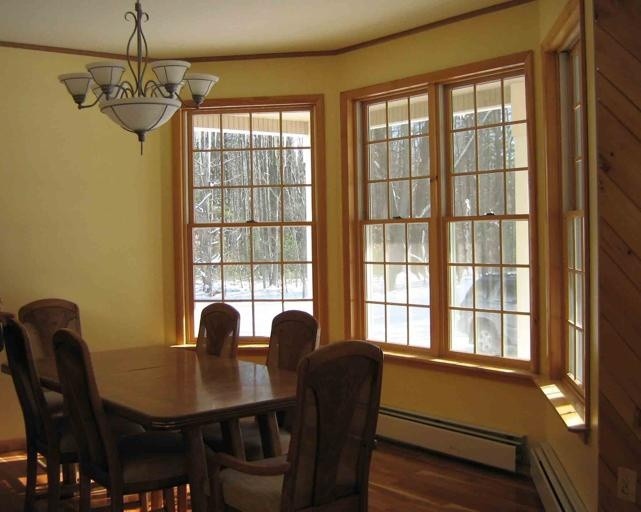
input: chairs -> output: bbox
[53,327,222,512]
[207,338,384,512]
[206,310,320,461]
[57,0,219,156]
[201,303,241,431]
[3,318,146,512]
[19,298,81,415]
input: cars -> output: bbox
[458,272,517,356]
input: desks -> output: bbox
[1,343,300,511]
